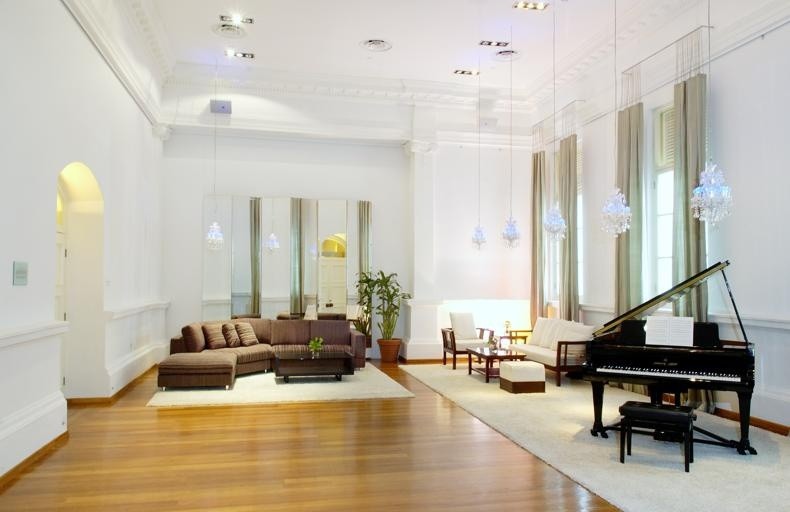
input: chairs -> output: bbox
[440,325,496,372]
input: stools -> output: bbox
[497,360,548,394]
[613,394,698,474]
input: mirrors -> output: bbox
[227,194,375,350]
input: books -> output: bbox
[644,317,694,347]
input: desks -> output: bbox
[498,334,528,348]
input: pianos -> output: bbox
[585,261,757,455]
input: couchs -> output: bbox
[156,315,367,391]
[509,328,595,388]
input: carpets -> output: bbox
[144,360,419,409]
[396,357,789,512]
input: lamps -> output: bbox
[540,0,571,245]
[690,0,735,227]
[263,194,283,253]
[472,1,491,248]
[500,0,524,248]
[597,0,636,238]
[202,60,231,252]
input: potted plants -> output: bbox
[350,270,372,348]
[370,269,413,363]
[484,335,499,350]
[308,334,324,360]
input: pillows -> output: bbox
[234,321,261,347]
[202,323,228,350]
[527,315,596,355]
[221,323,240,348]
[449,311,480,340]
